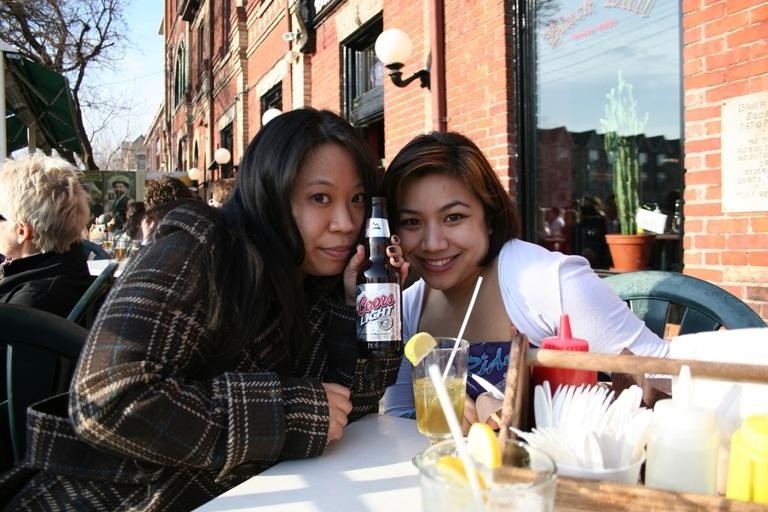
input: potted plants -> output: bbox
[599,71,656,271]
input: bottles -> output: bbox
[532,313,599,393]
[644,362,720,496]
[726,413,768,507]
[353,195,405,363]
[671,199,682,234]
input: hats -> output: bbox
[108,176,131,186]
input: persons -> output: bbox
[0,150,94,474]
[0,104,410,511]
[380,128,670,436]
[540,189,681,275]
[80,174,236,260]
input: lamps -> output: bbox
[187,27,430,188]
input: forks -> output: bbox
[507,380,653,471]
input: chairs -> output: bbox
[604,271,765,340]
[66,263,119,322]
[0,303,90,482]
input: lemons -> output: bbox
[404,332,438,367]
[437,421,503,504]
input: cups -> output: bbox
[408,336,471,438]
[103,240,139,261]
[411,434,558,512]
